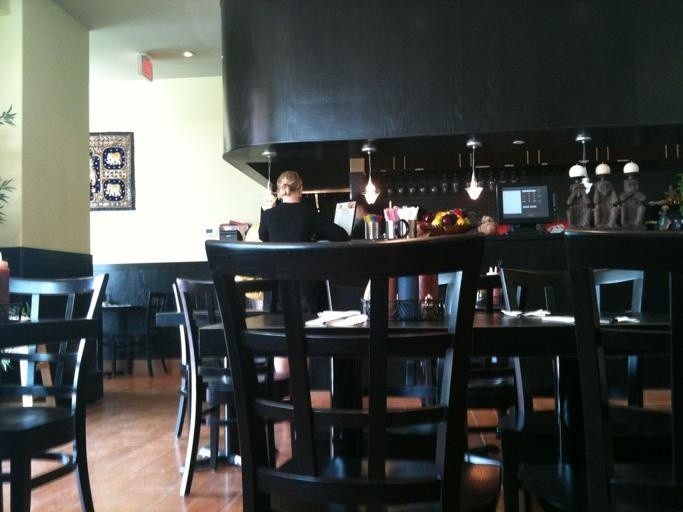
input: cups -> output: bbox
[364,220,410,241]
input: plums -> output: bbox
[442,215,452,226]
[422,213,433,222]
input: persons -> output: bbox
[344,192,384,238]
[257,170,349,399]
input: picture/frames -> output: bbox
[87,131,136,211]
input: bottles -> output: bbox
[381,170,521,194]
[565,175,645,229]
[362,273,437,321]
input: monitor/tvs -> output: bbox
[495,181,553,236]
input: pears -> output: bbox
[432,217,442,226]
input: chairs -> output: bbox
[173,230,683,512]
[110,292,173,377]
[1,273,110,512]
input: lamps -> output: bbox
[361,140,379,205]
[464,139,483,201]
[566,132,640,182]
[258,150,277,210]
